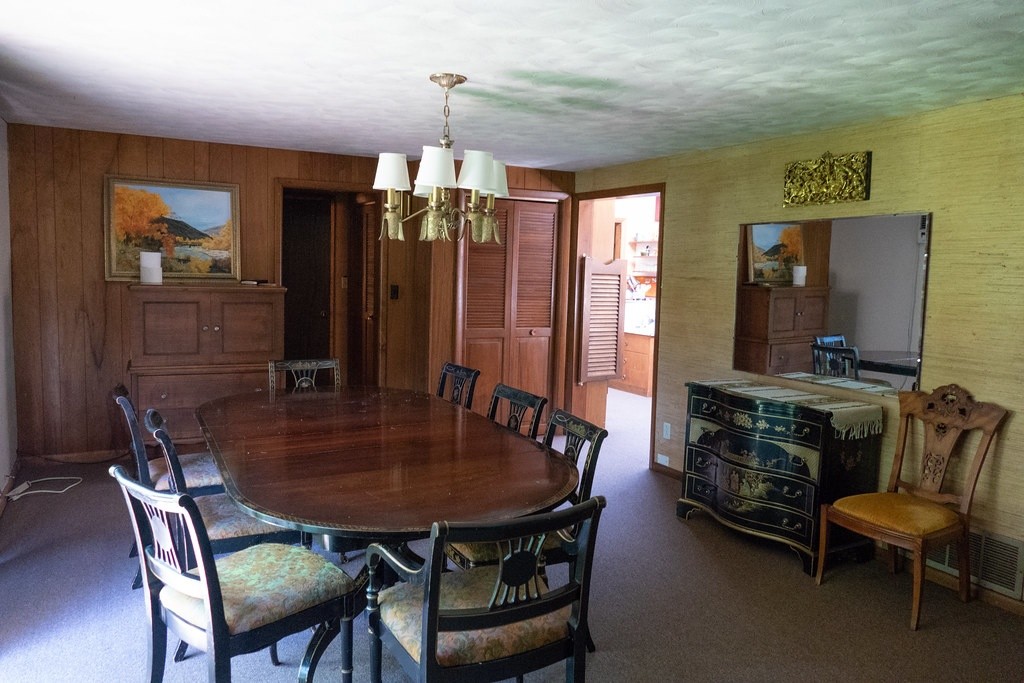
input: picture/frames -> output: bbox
[103,172,242,284]
[748,223,804,282]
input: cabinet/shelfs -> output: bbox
[733,220,832,375]
[608,241,657,398]
[677,380,884,581]
[125,282,287,445]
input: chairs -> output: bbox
[108,361,610,682]
[816,383,1009,632]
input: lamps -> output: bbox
[373,72,507,246]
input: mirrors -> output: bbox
[731,211,932,393]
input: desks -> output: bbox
[194,384,578,683]
